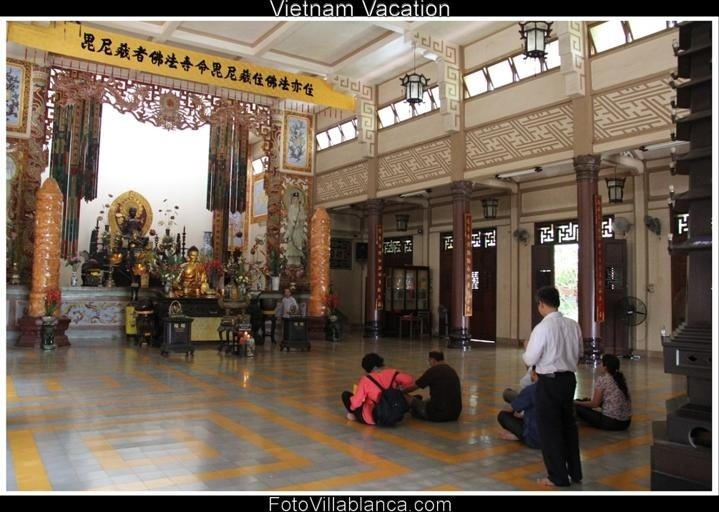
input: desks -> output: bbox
[261,315,278,344]
[280,316,312,352]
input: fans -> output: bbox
[612,217,632,237]
[614,295,647,360]
[513,228,532,246]
[643,214,663,235]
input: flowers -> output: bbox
[41,288,62,317]
[205,258,224,278]
[112,246,155,284]
[323,291,341,313]
[65,255,82,272]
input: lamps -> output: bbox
[518,21,554,59]
[395,212,410,232]
[605,165,627,203]
[400,44,430,105]
[482,194,499,220]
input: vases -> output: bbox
[124,284,140,335]
[71,272,78,287]
[42,316,57,349]
[327,313,343,341]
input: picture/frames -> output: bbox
[279,106,315,178]
[6,57,33,139]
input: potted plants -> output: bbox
[267,248,287,291]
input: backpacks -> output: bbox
[366,371,408,428]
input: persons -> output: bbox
[173,244,217,299]
[410,350,463,423]
[121,205,150,246]
[521,286,584,486]
[275,287,301,353]
[282,189,307,267]
[497,364,542,451]
[341,352,417,425]
[574,353,631,431]
